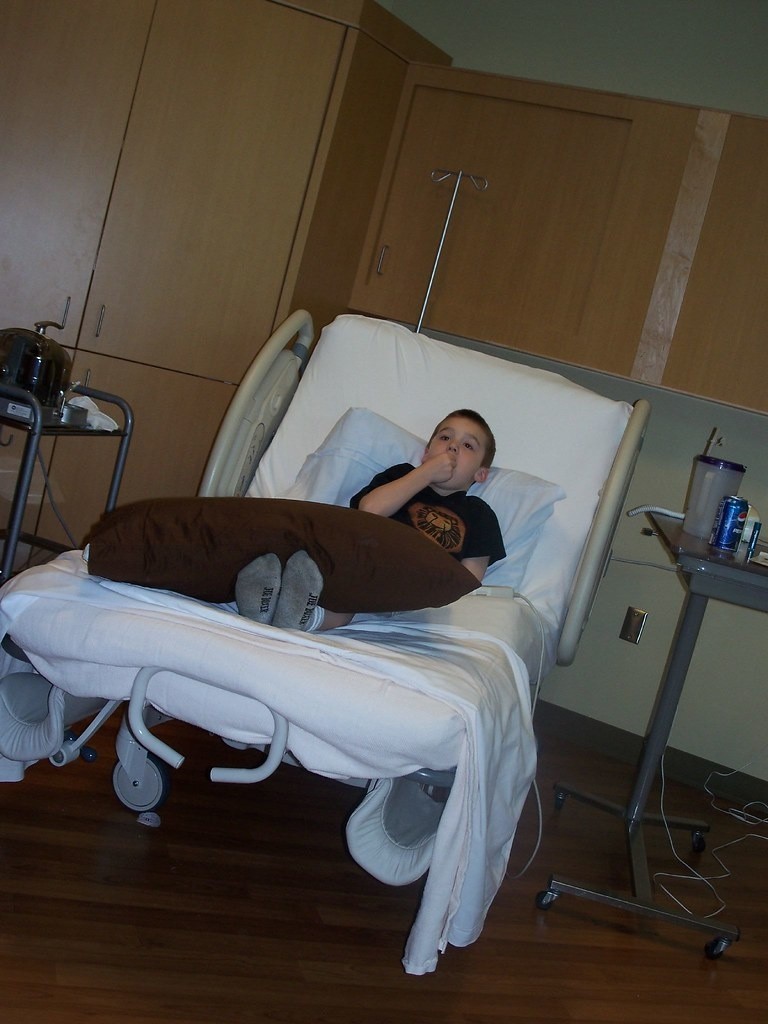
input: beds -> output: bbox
[0,309,650,975]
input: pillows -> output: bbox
[78,499,482,612]
[295,408,567,592]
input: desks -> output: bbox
[537,509,767,958]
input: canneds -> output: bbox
[708,495,749,552]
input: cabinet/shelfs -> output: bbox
[0,0,768,589]
[0,382,135,575]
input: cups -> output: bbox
[682,454,748,541]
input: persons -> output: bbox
[235,409,508,631]
